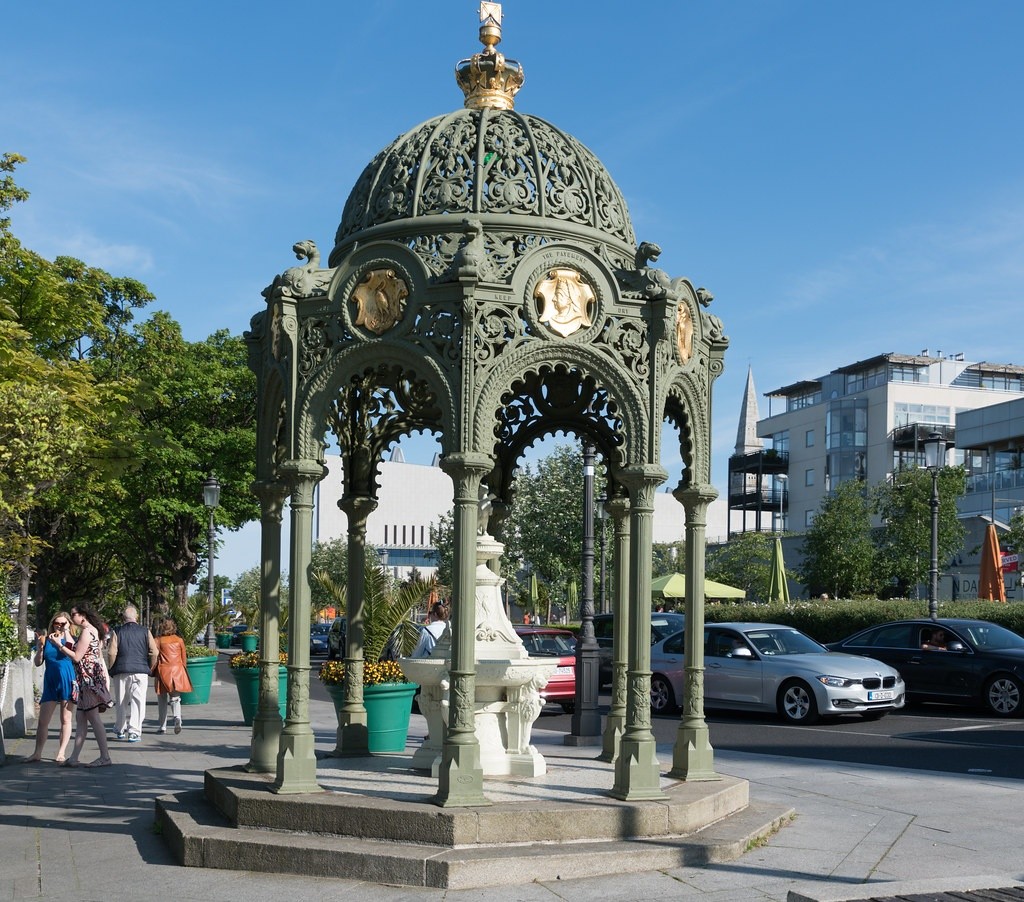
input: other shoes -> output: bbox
[157,730,165,734]
[174,717,181,734]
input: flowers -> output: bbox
[261,596,289,639]
[161,589,231,658]
[227,593,296,668]
[214,608,236,635]
[309,560,439,685]
[237,605,261,636]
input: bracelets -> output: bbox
[60,646,63,651]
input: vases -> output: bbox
[280,637,288,652]
[242,635,257,652]
[218,634,231,648]
[231,667,288,724]
[178,654,219,703]
[326,682,419,752]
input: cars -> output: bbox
[576,613,685,692]
[650,623,906,726]
[230,625,247,646]
[310,623,331,655]
[327,617,346,661]
[514,627,579,714]
[824,619,1024,718]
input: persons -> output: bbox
[19,611,76,763]
[820,594,828,601]
[922,629,947,650]
[153,617,193,734]
[49,601,116,768]
[411,598,452,743]
[655,605,663,612]
[523,611,535,624]
[552,613,556,625]
[107,607,159,742]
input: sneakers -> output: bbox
[128,733,140,742]
[114,727,125,738]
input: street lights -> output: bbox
[922,426,949,618]
[379,548,390,593]
[205,472,221,651]
[775,474,788,535]
[595,491,610,614]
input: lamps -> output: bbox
[952,553,963,566]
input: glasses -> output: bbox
[70,612,78,618]
[53,622,65,626]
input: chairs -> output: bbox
[542,639,557,650]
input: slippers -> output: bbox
[84,759,111,767]
[21,756,40,763]
[59,758,78,767]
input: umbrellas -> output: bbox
[978,522,1005,603]
[524,570,539,616]
[766,538,790,604]
[427,580,438,610]
[567,571,578,609]
[651,572,746,608]
